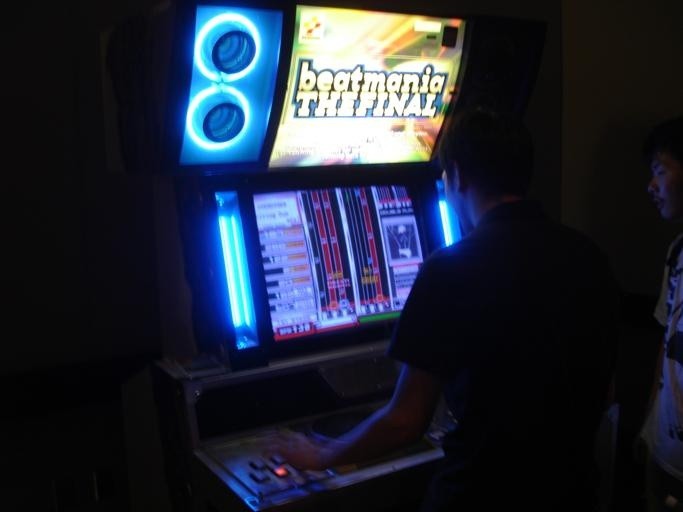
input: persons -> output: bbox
[630,116,682,510]
[269,106,642,510]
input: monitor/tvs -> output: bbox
[254,185,423,341]
